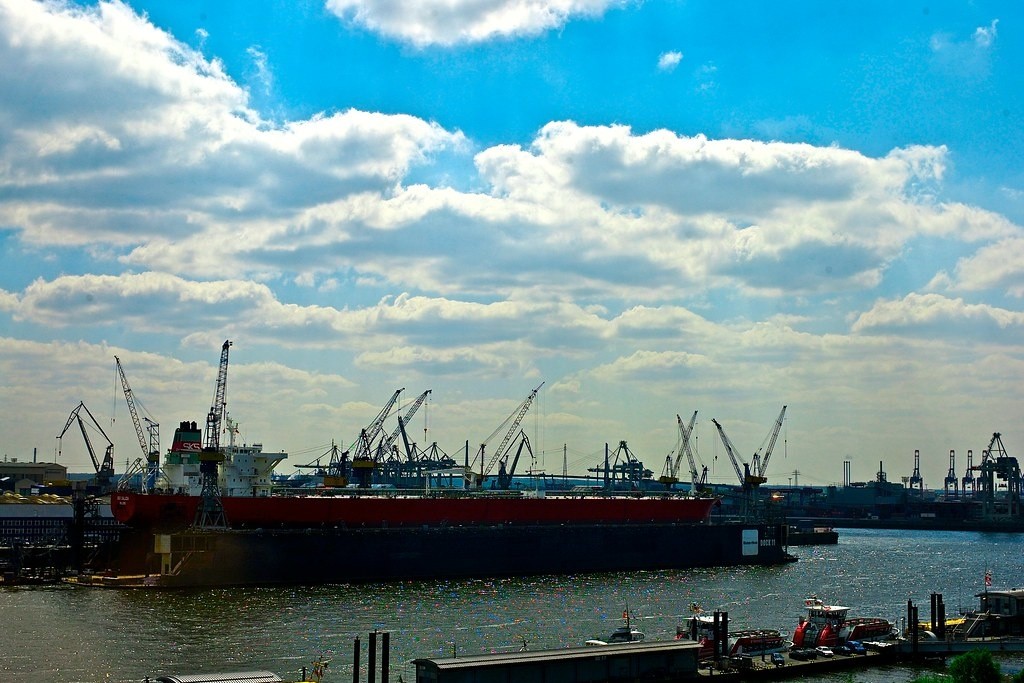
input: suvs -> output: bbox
[847,641,866,655]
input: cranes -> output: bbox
[290,387,432,488]
[108,338,241,554]
[458,381,544,493]
[712,403,789,525]
[55,401,116,500]
[658,409,711,498]
[907,432,1024,499]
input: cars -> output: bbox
[771,652,787,667]
[832,646,852,657]
[790,647,818,660]
[816,646,834,658]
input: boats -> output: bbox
[790,596,900,653]
[586,601,646,649]
[674,601,793,664]
[905,567,1024,641]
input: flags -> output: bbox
[985,567,994,586]
